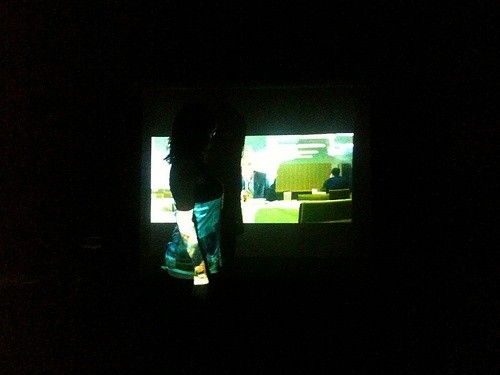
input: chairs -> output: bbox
[329,188,351,199]
[298,200,352,223]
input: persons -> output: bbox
[161,101,223,298]
[323,168,345,193]
